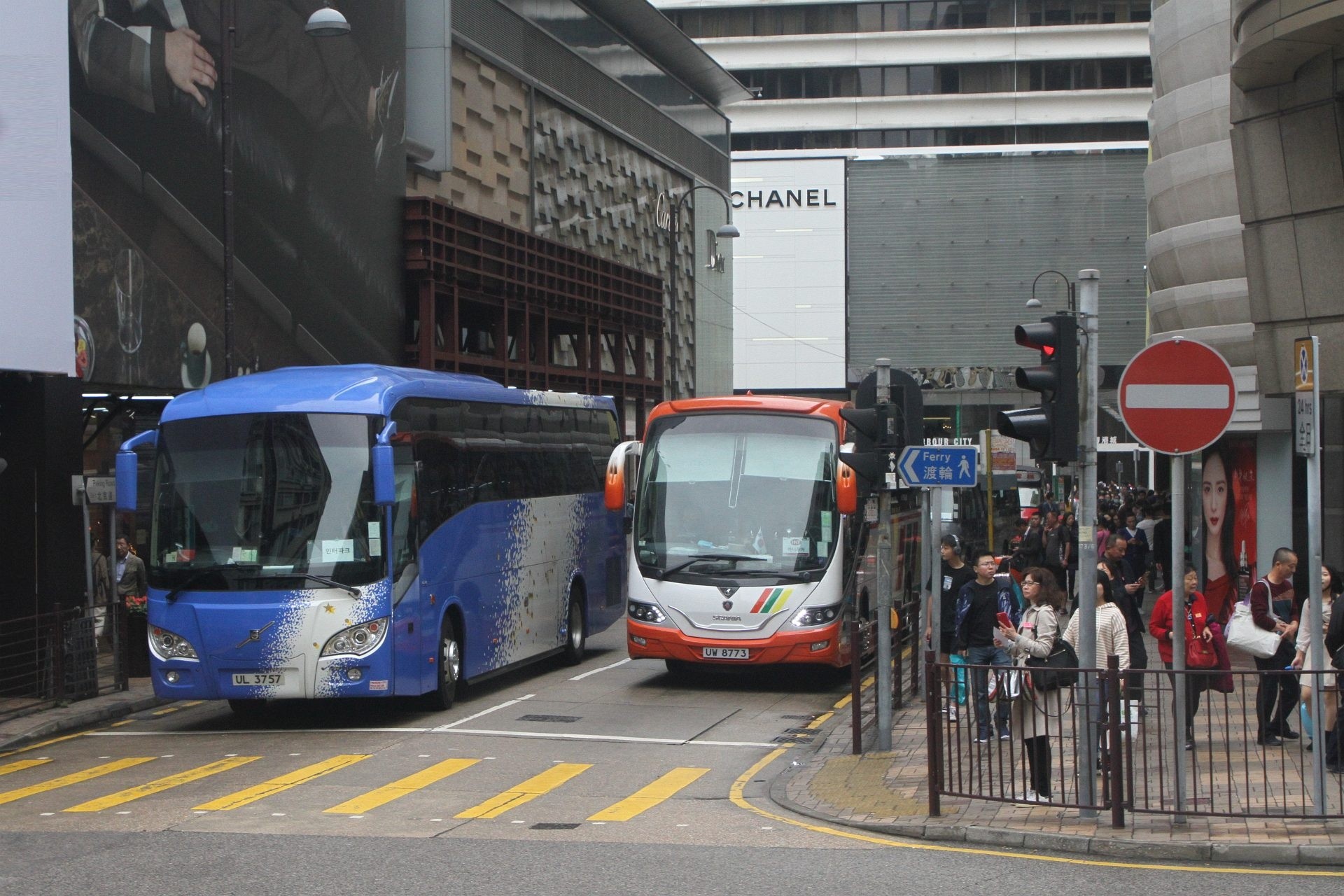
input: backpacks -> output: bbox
[88,553,103,605]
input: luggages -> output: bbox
[1284,666,1313,741]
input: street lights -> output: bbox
[669,182,742,400]
[1026,269,1079,514]
[217,0,354,377]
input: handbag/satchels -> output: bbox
[987,673,997,704]
[1145,550,1156,571]
[1025,604,1080,692]
[1223,578,1283,659]
[1119,699,1139,742]
[1057,525,1071,566]
[1187,637,1219,668]
[949,654,970,707]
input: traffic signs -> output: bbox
[896,445,979,487]
[86,476,115,504]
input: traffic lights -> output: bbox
[995,312,1078,469]
[837,403,906,492]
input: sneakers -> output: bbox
[1077,758,1101,777]
[1010,786,1036,805]
[1016,789,1053,808]
[949,706,956,722]
[1101,759,1111,780]
[973,732,995,743]
[942,702,947,713]
[1000,727,1010,741]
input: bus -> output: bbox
[114,364,633,715]
[604,388,920,686]
[926,465,1048,566]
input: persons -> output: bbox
[626,490,636,507]
[69,0,404,321]
[1200,447,1239,627]
[90,536,151,676]
[925,481,1344,808]
[1149,561,1234,749]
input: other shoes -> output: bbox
[1257,738,1281,746]
[1185,738,1192,750]
[1307,743,1313,751]
[1272,728,1299,739]
[1139,606,1147,614]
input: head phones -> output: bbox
[952,534,962,555]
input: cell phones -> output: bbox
[997,612,1011,628]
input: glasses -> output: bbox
[976,563,997,567]
[1021,580,1041,586]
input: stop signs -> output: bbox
[1115,336,1239,457]
